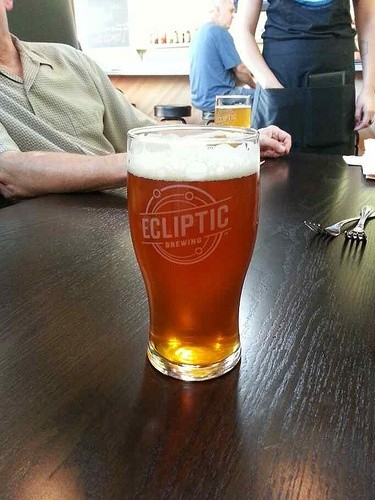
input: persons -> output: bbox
[0,0,291,210]
[190,0,257,113]
[233,0,375,148]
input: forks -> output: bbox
[304,211,375,237]
[344,205,373,240]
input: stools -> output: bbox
[154,105,191,124]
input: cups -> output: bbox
[126,125,260,381]
[214,95,251,128]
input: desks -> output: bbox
[0,153,375,500]
